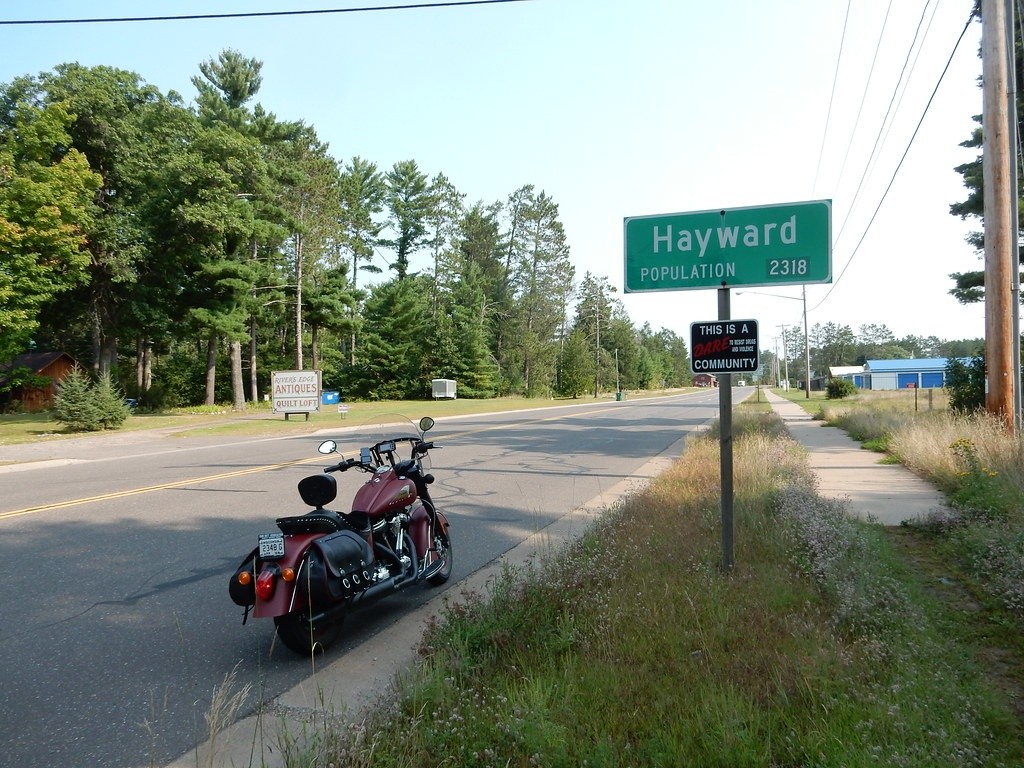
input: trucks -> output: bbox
[737,381,745,387]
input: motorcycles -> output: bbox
[252,412,453,659]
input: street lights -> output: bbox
[735,290,810,399]
